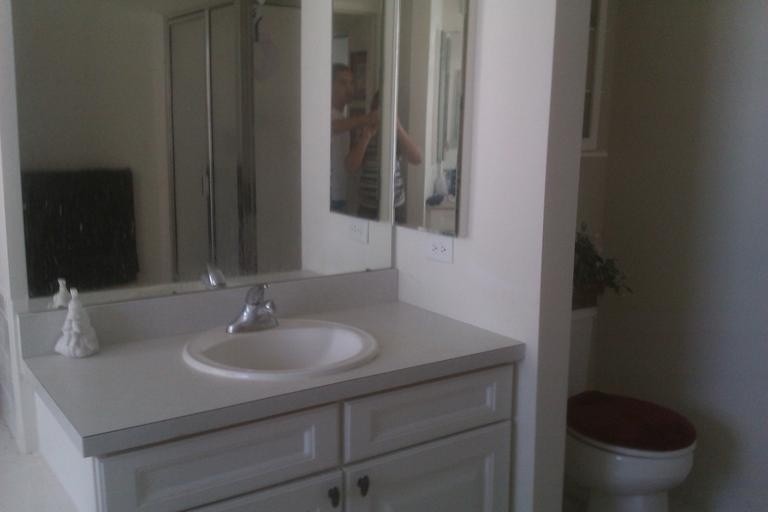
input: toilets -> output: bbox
[566,302,701,511]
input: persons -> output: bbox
[331,62,380,222]
[342,92,423,226]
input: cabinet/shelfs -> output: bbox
[97,361,516,511]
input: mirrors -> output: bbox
[393,1,470,238]
[12,1,395,313]
[328,1,392,221]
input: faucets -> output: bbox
[202,256,228,288]
[222,279,280,332]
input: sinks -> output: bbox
[181,313,380,383]
